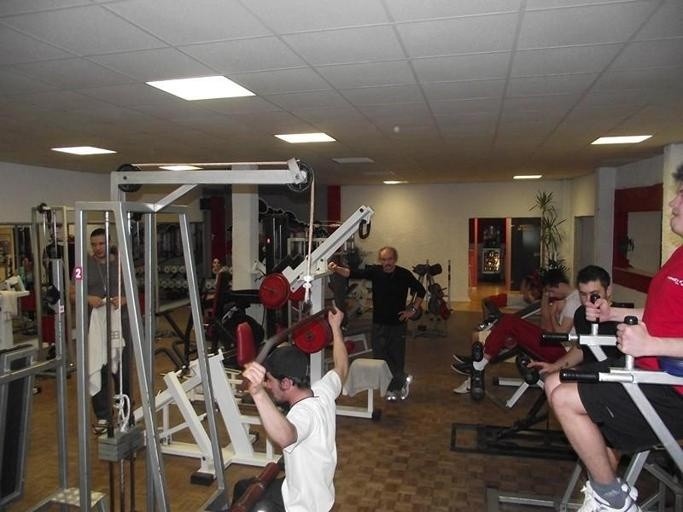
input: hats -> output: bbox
[260,345,310,384]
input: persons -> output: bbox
[328,247,426,401]
[65,227,132,434]
[541,161,683,511]
[449,269,583,375]
[15,256,42,282]
[208,255,233,289]
[526,264,632,375]
[231,308,348,512]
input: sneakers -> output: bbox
[453,353,474,363]
[575,479,642,512]
[450,362,474,375]
[92,419,109,435]
[400,373,413,400]
[453,377,471,394]
[385,390,398,401]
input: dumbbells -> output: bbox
[135,265,213,290]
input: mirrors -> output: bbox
[610,184,663,294]
[0,221,117,333]
